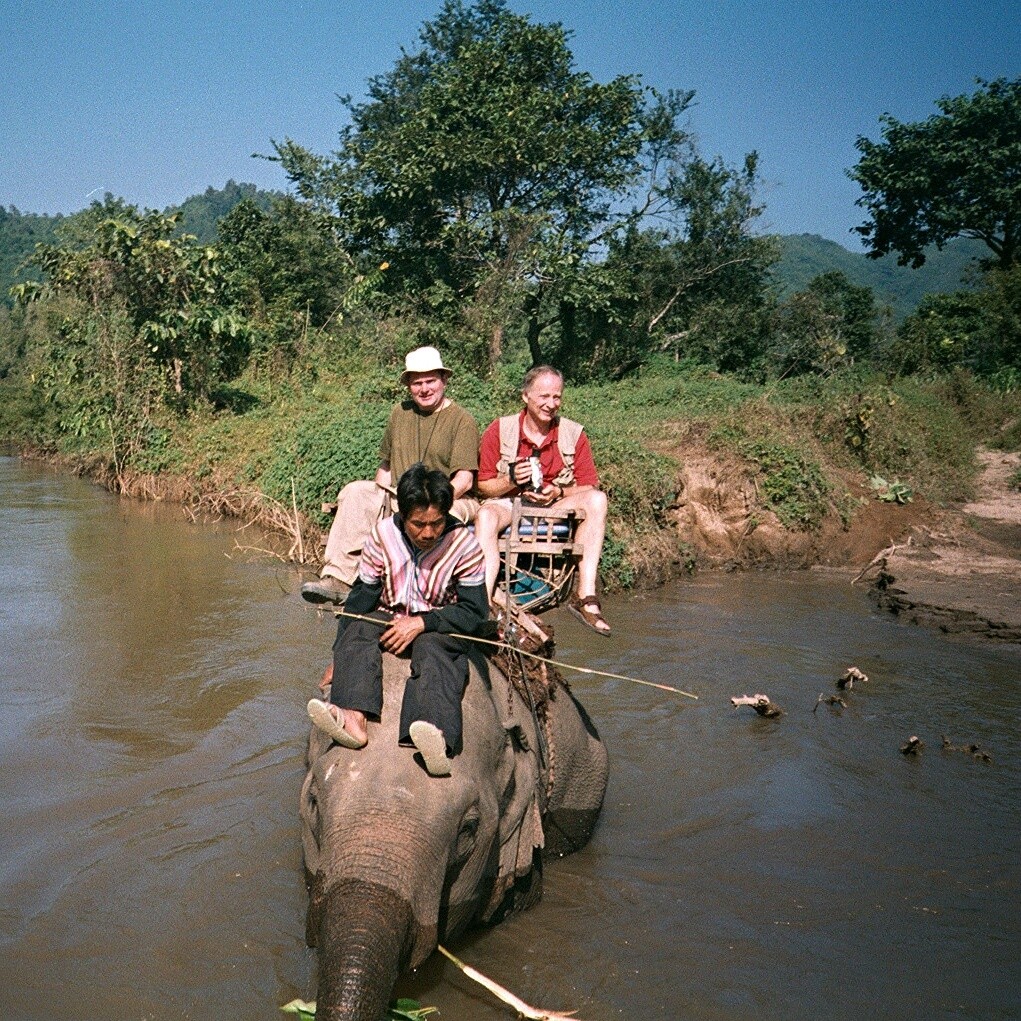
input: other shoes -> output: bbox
[301,575,352,606]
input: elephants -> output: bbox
[298,607,610,1021]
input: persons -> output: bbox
[300,342,479,605]
[477,366,612,638]
[307,462,489,775]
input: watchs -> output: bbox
[553,486,564,501]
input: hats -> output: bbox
[401,346,454,385]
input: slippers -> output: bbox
[307,698,360,749]
[409,720,452,777]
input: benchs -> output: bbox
[465,496,583,555]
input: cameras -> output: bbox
[525,456,544,494]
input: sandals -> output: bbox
[567,591,612,637]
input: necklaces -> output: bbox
[418,399,446,461]
